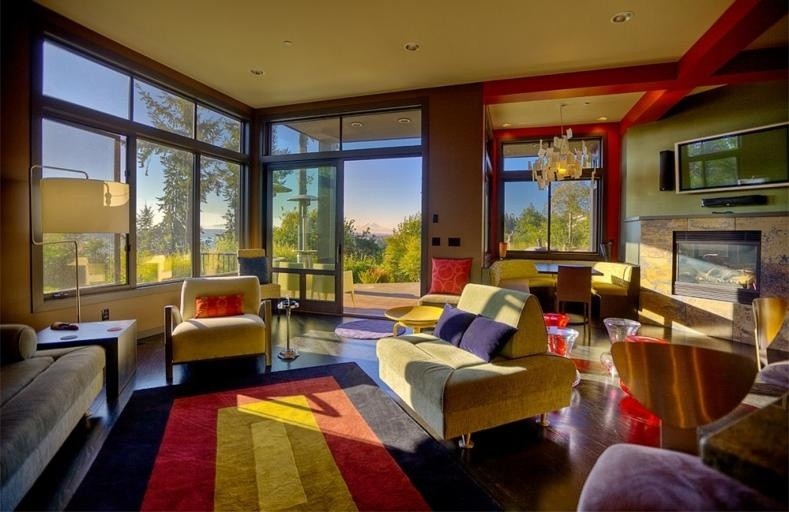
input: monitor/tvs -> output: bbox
[675,121,789,195]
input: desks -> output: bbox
[699,392,789,501]
[533,263,605,291]
[383,305,444,333]
[36,317,139,416]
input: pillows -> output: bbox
[427,255,473,295]
[459,314,520,362]
[433,304,477,348]
[193,291,246,319]
[237,256,270,284]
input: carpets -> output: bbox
[64,359,505,510]
[334,318,406,340]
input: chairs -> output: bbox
[751,296,789,373]
[313,263,356,307]
[163,275,272,385]
[375,282,577,448]
[277,261,312,298]
[237,249,282,320]
[553,264,592,346]
[609,340,759,454]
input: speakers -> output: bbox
[659,150,674,191]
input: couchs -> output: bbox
[0,323,113,511]
[488,258,641,321]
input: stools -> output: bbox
[418,294,461,307]
[545,325,583,389]
[620,333,672,401]
[543,313,571,355]
[601,317,641,377]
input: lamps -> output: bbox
[528,102,601,191]
[29,164,131,323]
